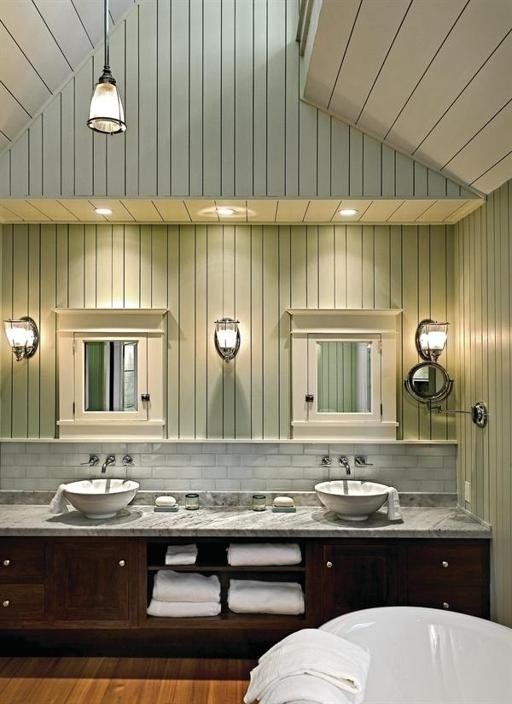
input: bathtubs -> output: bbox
[319,604,512,704]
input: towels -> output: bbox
[383,487,402,520]
[227,542,302,565]
[242,627,371,703]
[152,569,221,601]
[165,544,198,566]
[228,578,304,614]
[49,483,70,515]
[145,599,223,617]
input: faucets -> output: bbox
[100,456,116,474]
[338,454,351,475]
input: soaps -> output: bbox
[154,494,177,507]
[272,496,295,507]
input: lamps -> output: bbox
[419,320,448,362]
[86,0,128,134]
[3,317,40,361]
[213,318,241,362]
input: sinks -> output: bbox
[314,479,390,522]
[63,478,141,520]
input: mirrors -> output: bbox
[289,308,402,440]
[55,309,166,439]
[406,364,487,427]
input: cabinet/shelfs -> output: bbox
[47,537,147,636]
[147,538,315,645]
[320,539,406,628]
[407,539,489,619]
[0,536,46,636]
